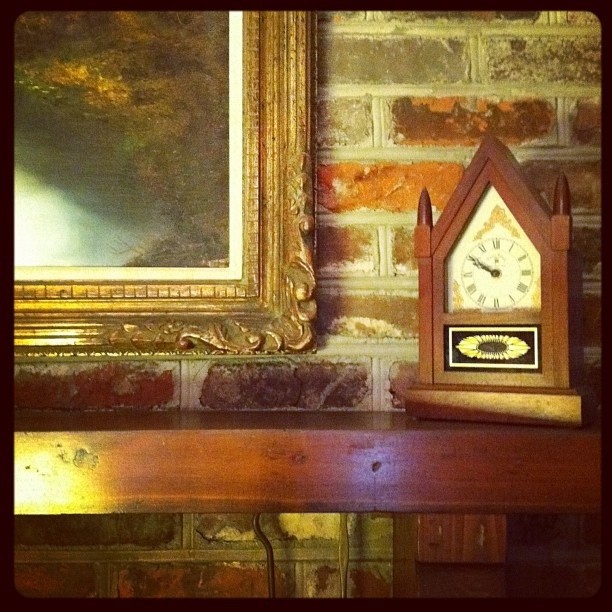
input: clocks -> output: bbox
[389,136,602,426]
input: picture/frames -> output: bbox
[12,8,317,356]
